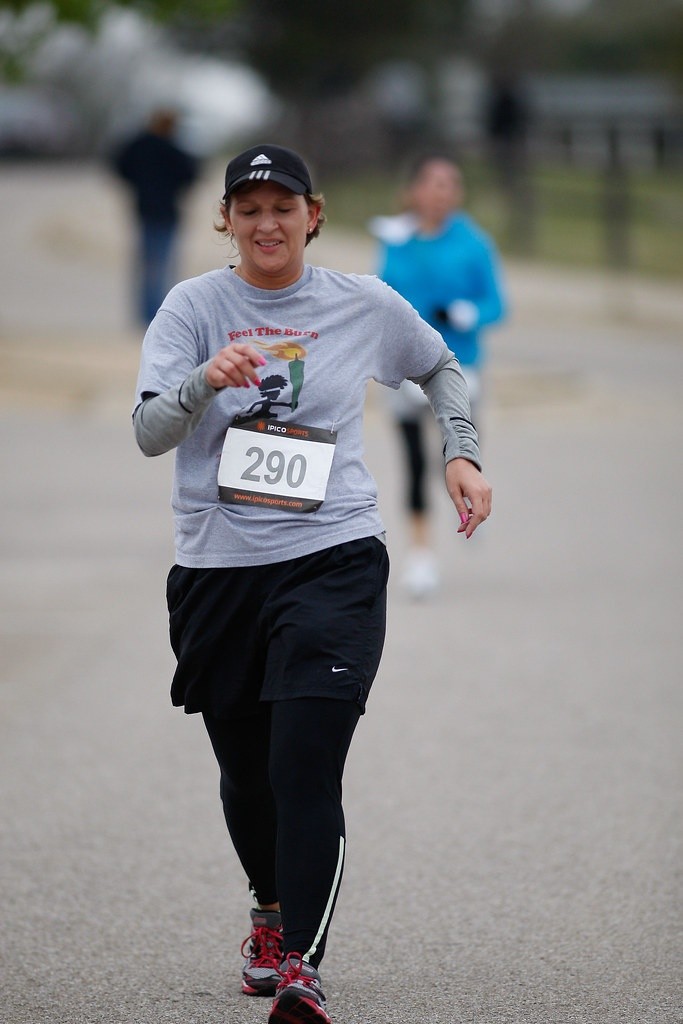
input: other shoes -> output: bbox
[406,549,439,596]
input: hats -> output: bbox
[220,144,312,195]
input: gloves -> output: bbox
[447,297,474,330]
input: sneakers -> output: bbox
[269,952,332,1024]
[240,907,282,996]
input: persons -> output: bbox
[373,151,508,544]
[128,142,496,1024]
[111,109,205,329]
